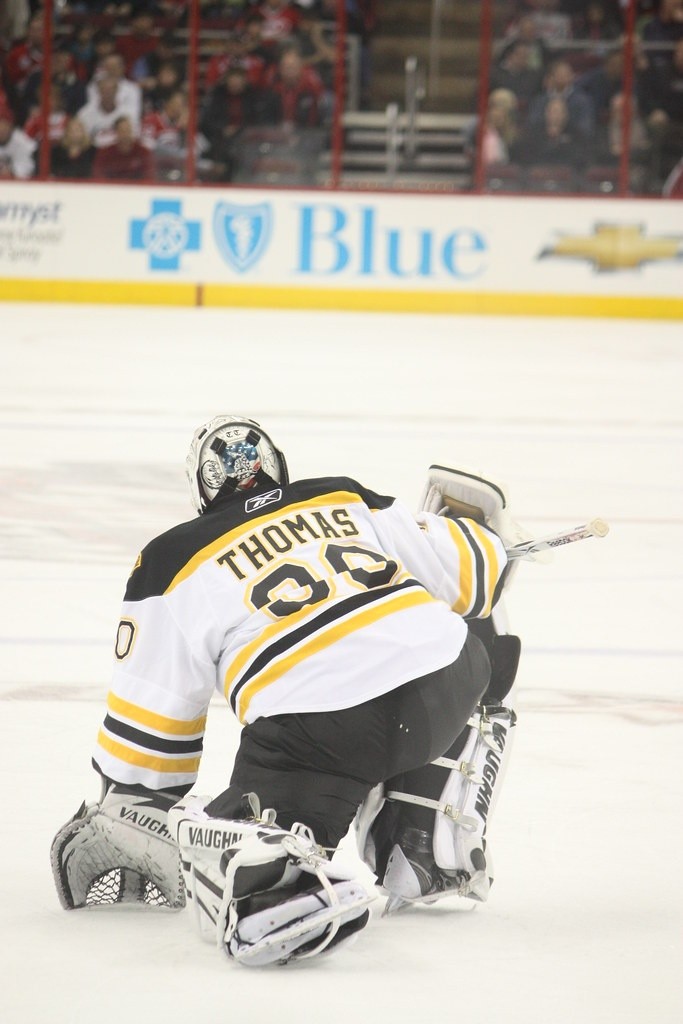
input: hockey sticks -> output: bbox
[496,517,608,558]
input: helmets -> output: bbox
[184,414,290,515]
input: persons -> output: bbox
[0,0,374,188]
[51,414,520,965]
[457,0,683,195]
